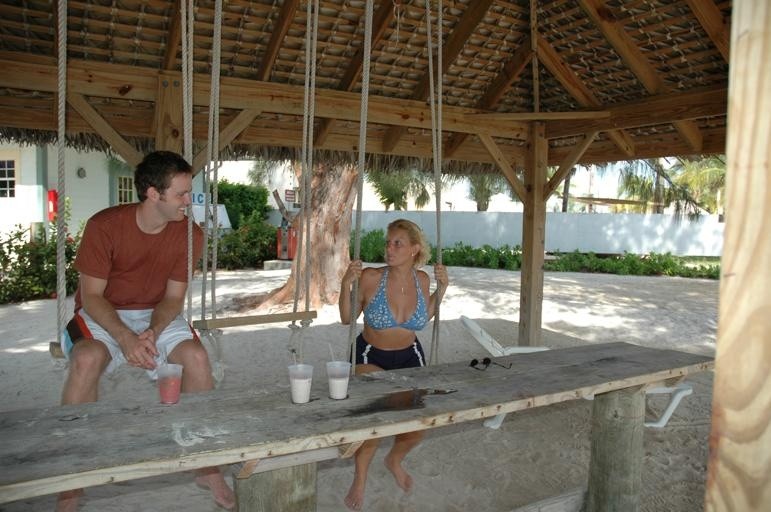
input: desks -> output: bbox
[0,341,718,511]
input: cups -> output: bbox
[325,361,352,400]
[156,363,184,405]
[288,364,315,404]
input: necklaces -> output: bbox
[392,271,412,296]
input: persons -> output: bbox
[53,150,238,512]
[339,218,448,510]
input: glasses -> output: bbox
[470,358,512,371]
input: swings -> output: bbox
[48,0,192,357]
[193,0,318,330]
[346,0,443,373]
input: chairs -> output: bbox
[459,314,695,431]
